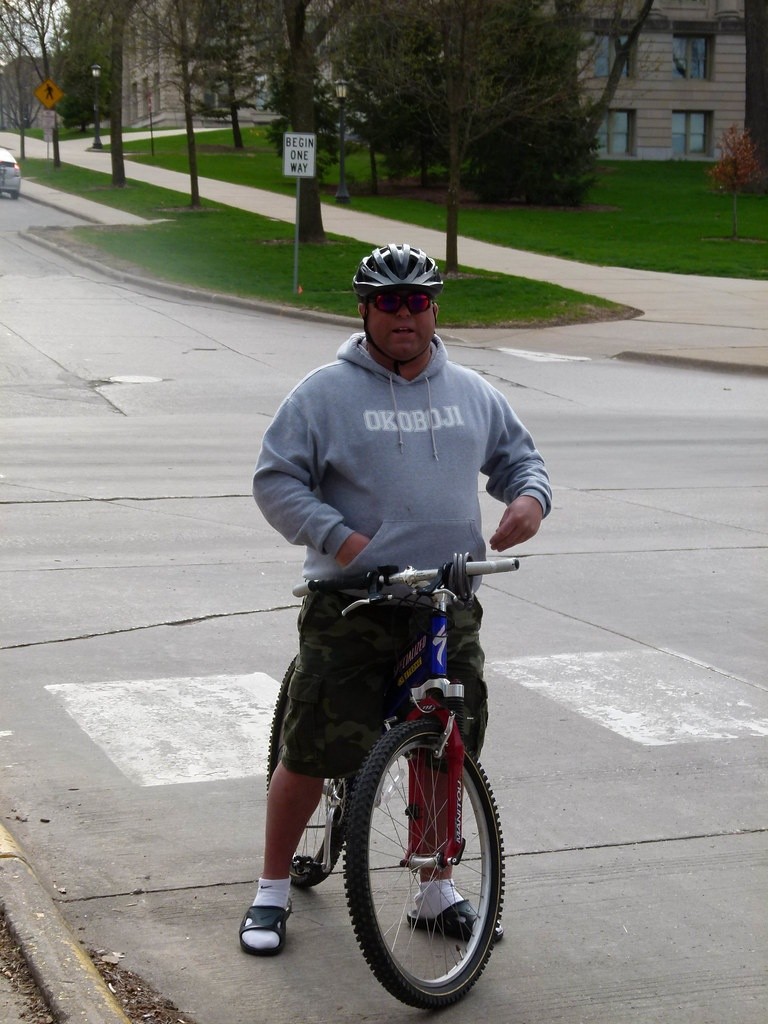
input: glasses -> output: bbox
[368,291,433,314]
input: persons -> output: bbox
[237,242,553,958]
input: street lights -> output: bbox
[334,75,350,205]
[90,62,103,148]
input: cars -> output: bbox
[0,145,21,199]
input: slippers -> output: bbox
[239,897,292,956]
[407,899,503,944]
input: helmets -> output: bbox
[351,243,445,298]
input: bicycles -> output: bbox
[267,560,521,1010]
